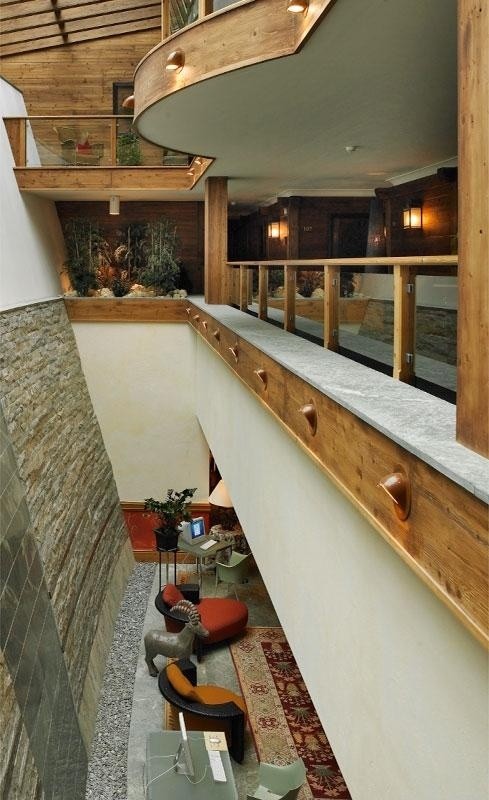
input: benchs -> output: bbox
[252,268,370,325]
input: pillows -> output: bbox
[162,584,184,607]
[165,663,207,704]
[78,141,93,155]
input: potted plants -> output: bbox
[117,129,142,166]
[145,488,198,549]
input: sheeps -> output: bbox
[144,600,210,677]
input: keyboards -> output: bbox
[200,539,217,551]
[208,750,228,784]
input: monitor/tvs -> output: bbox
[178,516,206,546]
[173,712,195,778]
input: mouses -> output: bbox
[209,737,220,743]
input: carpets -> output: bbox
[224,624,352,800]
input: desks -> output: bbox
[145,728,240,800]
[176,523,247,592]
[154,544,180,594]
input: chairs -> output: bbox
[156,580,248,661]
[213,552,253,601]
[53,125,104,167]
[247,757,307,800]
[160,658,248,764]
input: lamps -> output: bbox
[286,0,308,17]
[377,464,412,523]
[271,216,280,238]
[121,95,134,110]
[254,369,267,390]
[165,51,184,74]
[207,477,237,531]
[401,198,422,231]
[298,397,318,436]
[108,195,121,215]
